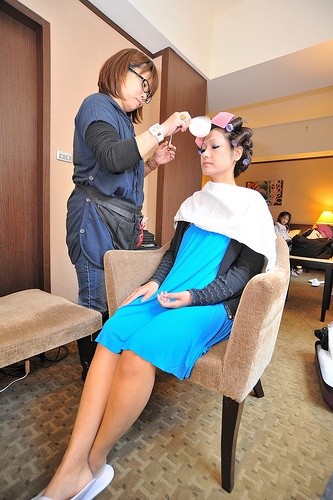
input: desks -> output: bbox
[288,255,333,323]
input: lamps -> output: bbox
[316,211,333,225]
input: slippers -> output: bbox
[30,464,114,499]
[38,478,96,499]
[309,278,325,284]
[311,281,320,286]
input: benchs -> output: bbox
[0,288,102,382]
[285,223,333,242]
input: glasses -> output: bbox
[128,67,152,104]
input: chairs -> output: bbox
[103,233,290,493]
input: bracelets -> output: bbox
[147,158,156,170]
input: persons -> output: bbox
[65,48,191,383]
[31,112,277,500]
[274,212,292,252]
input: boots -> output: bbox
[76,309,109,382]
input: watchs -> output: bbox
[148,123,165,142]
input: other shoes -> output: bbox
[291,269,298,277]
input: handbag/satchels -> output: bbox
[287,234,333,259]
